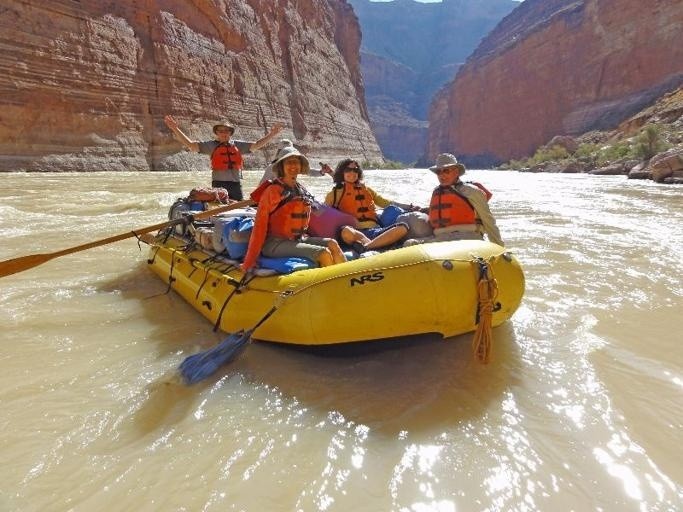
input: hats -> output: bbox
[278,139,293,150]
[430,153,465,176]
[272,146,310,176]
[213,119,234,136]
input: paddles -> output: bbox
[0,198,257,278]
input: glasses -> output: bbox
[344,166,359,172]
[436,169,450,175]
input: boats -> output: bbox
[146,199,526,347]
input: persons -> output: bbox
[239,147,348,272]
[403,152,505,248]
[163,113,283,201]
[324,158,421,254]
[258,138,331,185]
